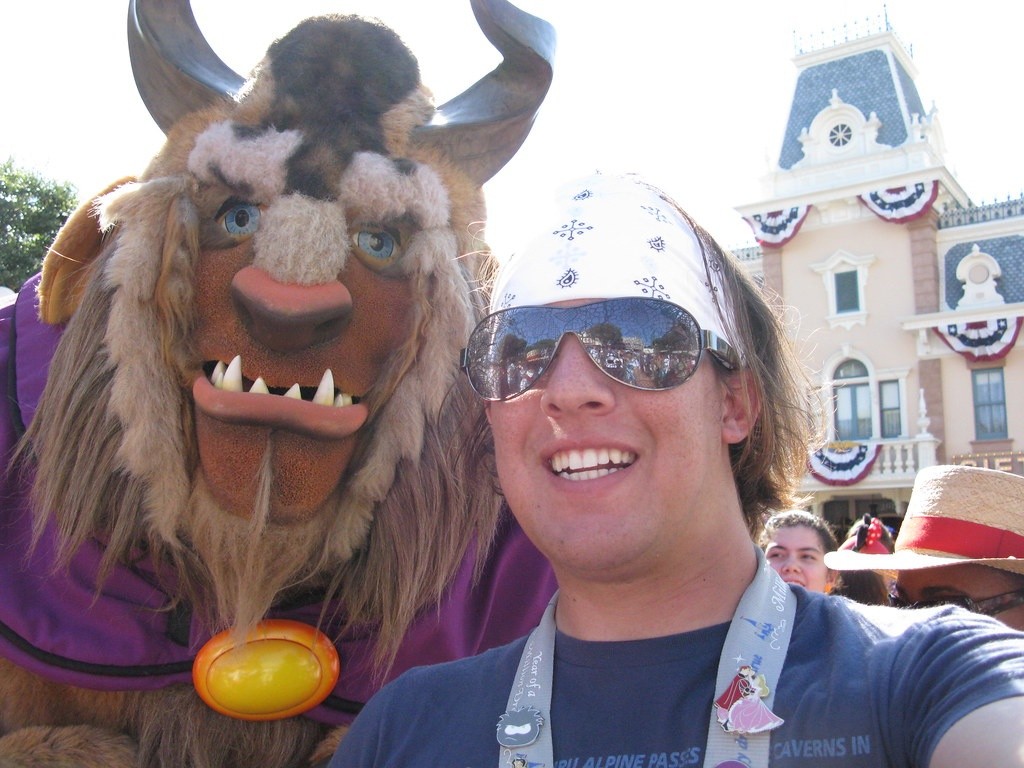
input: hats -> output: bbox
[823,464,1024,575]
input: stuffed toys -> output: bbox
[0,0,557,768]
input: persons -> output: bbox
[327,182,1024,768]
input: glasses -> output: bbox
[459,295,740,402]
